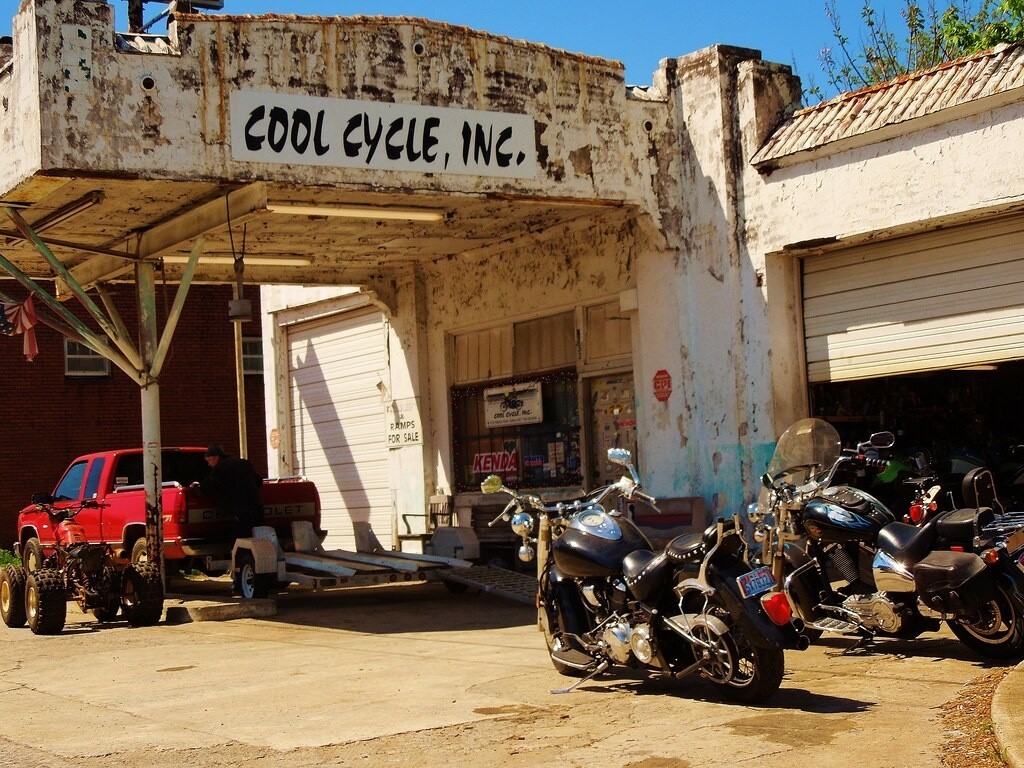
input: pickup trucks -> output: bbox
[16,446,323,602]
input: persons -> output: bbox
[187,445,267,549]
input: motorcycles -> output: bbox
[482,447,810,708]
[0,491,165,637]
[730,416,1024,665]
[899,435,947,527]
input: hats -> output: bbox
[204,444,224,456]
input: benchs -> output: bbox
[470,498,587,549]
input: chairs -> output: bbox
[622,497,708,548]
[395,494,454,555]
